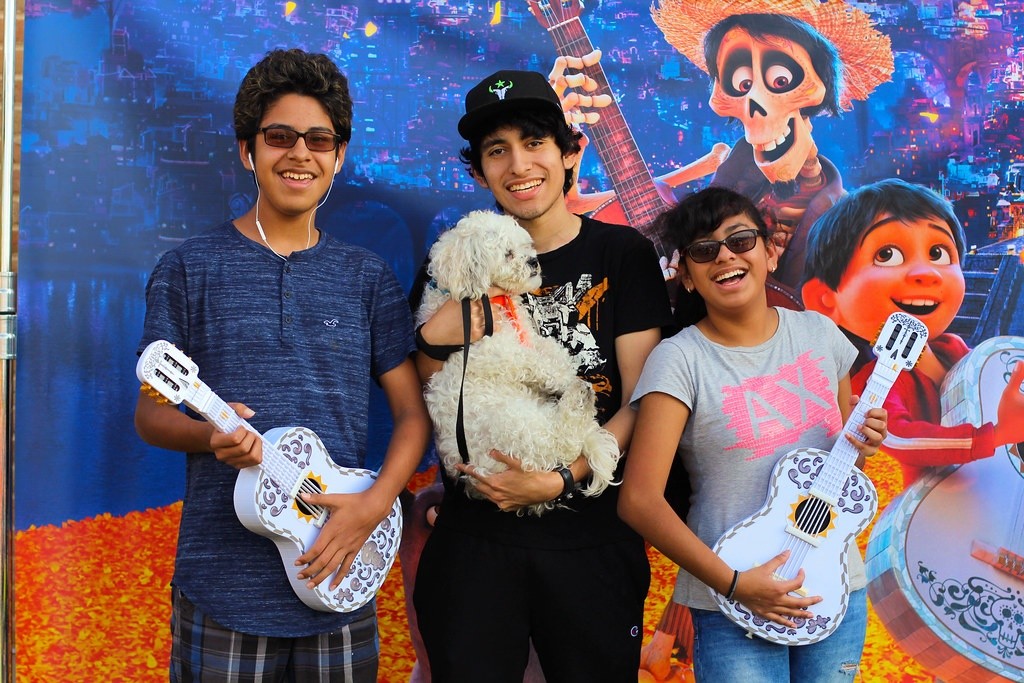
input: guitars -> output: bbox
[705,311,931,646]
[136,340,404,613]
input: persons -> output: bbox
[407,70,673,683]
[133,49,429,683]
[614,186,888,683]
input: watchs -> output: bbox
[551,466,578,503]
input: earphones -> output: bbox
[248,152,255,171]
[334,157,338,174]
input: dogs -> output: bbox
[414,210,621,517]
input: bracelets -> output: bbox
[725,570,739,601]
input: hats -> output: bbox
[457,70,564,142]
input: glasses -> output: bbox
[257,127,341,153]
[684,229,764,263]
